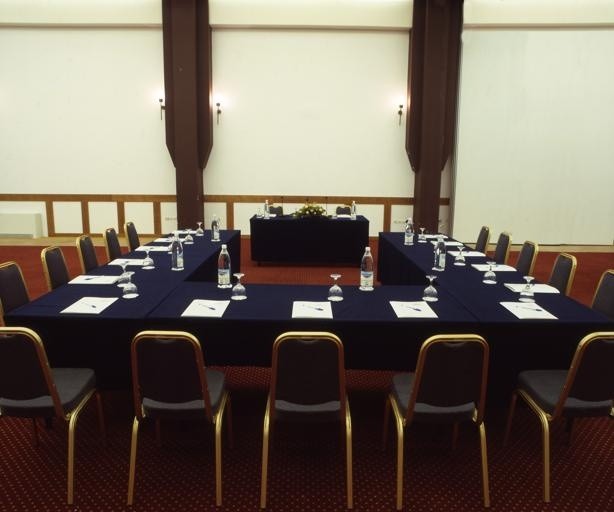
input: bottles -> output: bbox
[171,232,184,271]
[350,201,356,221]
[263,200,270,220]
[359,247,374,291]
[211,214,220,243]
[431,236,446,271]
[404,217,414,246]
[217,244,232,288]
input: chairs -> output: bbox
[0,261,32,314]
[386,333,491,508]
[548,251,577,295]
[124,221,140,253]
[476,226,491,255]
[41,244,70,291]
[260,332,353,508]
[269,206,283,214]
[336,206,351,214]
[591,269,614,323]
[514,240,539,277]
[76,234,99,274]
[1,326,106,503]
[127,330,233,506]
[504,331,614,503]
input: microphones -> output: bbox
[325,196,328,216]
[281,196,284,216]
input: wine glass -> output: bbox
[256,208,262,218]
[231,273,247,300]
[482,261,496,284]
[422,275,438,301]
[117,261,129,287]
[141,247,155,269]
[454,246,466,265]
[328,274,343,301]
[332,210,337,219]
[418,228,427,243]
[122,272,139,299]
[184,229,194,244]
[518,276,536,302]
[195,222,204,236]
[434,235,448,253]
[168,232,174,254]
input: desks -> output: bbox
[147,279,480,373]
[250,215,369,264]
[3,230,241,379]
[377,232,613,408]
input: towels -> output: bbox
[504,282,560,294]
[291,301,333,321]
[181,299,231,318]
[59,296,119,314]
[426,234,519,273]
[499,301,559,320]
[389,299,438,319]
[108,229,197,266]
[68,275,120,285]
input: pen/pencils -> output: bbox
[202,304,216,310]
[303,304,324,310]
[410,307,421,311]
[521,306,543,311]
[85,275,104,280]
[80,300,96,308]
